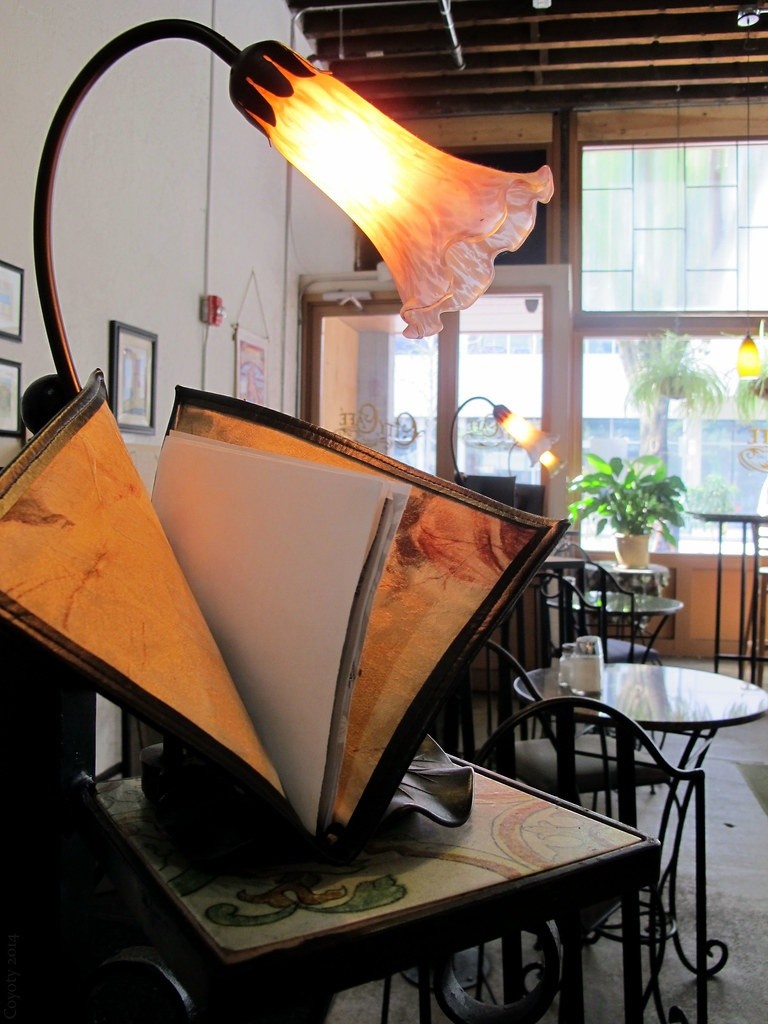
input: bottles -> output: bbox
[569,636,603,697]
[558,643,574,687]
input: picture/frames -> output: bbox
[109,320,158,436]
[234,325,269,409]
[0,358,22,438]
[0,260,24,345]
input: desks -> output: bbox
[546,590,684,751]
[87,751,661,1024]
[510,661,767,978]
[583,560,670,647]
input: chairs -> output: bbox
[378,543,708,1024]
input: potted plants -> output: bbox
[566,448,687,569]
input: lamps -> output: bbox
[737,310,763,377]
[21,20,554,867]
[450,396,560,488]
[508,437,567,490]
